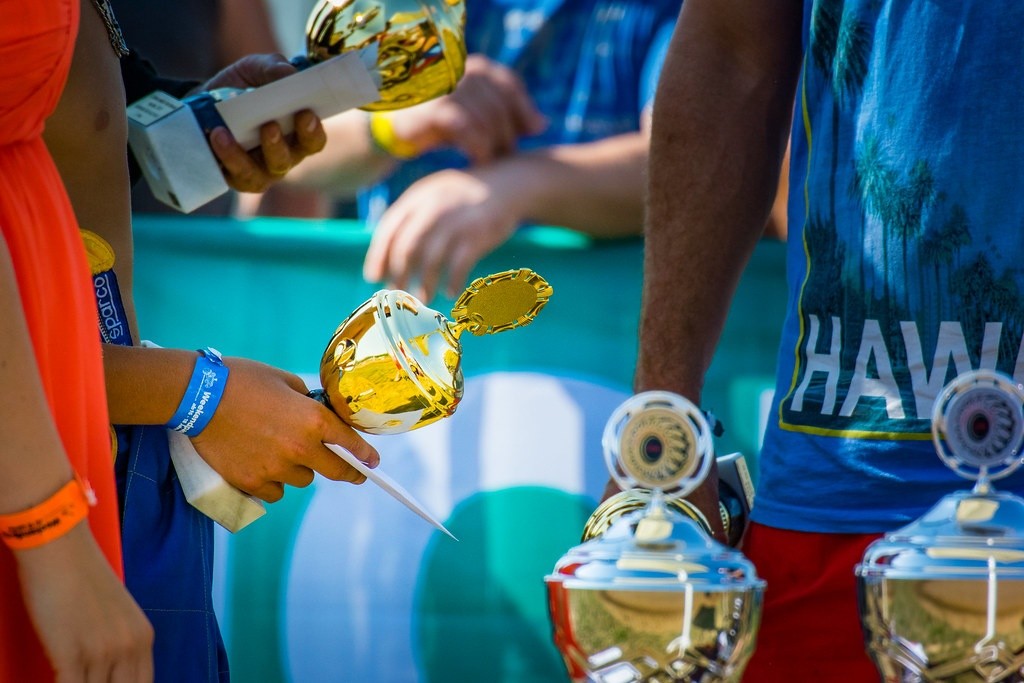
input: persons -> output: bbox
[598,1,1024,683]
[1,0,380,681]
[113,1,326,222]
[237,0,798,303]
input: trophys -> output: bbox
[126,0,467,214]
[853,369,1024,682]
[542,390,767,683]
[169,270,553,532]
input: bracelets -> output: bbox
[368,109,416,162]
[0,473,97,549]
[166,346,229,439]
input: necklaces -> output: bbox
[93,0,129,58]
